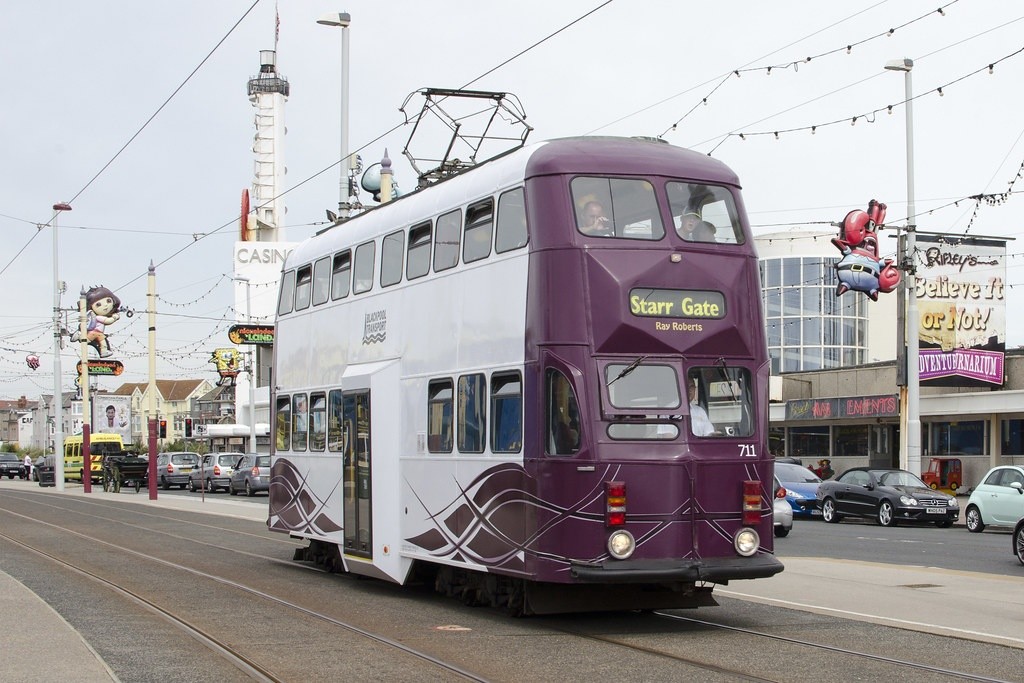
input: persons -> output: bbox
[676,205,717,242]
[657,374,722,438]
[567,421,578,446]
[70,285,121,358]
[505,431,521,451]
[105,405,116,428]
[579,202,610,237]
[22,451,32,480]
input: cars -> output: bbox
[33,456,46,482]
[145,452,202,489]
[964,465,1024,532]
[229,453,270,497]
[0,452,25,479]
[921,457,962,491]
[814,467,961,527]
[773,462,823,514]
[772,478,793,538]
[188,452,245,494]
[1012,516,1024,566]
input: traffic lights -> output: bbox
[158,419,167,439]
[184,418,193,438]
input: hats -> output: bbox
[680,205,702,219]
[577,193,604,210]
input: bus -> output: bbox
[264,135,784,619]
[63,434,124,484]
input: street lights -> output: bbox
[233,277,257,453]
[52,201,73,490]
[882,56,922,486]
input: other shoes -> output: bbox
[26,478,31,481]
[22,476,25,481]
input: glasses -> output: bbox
[687,384,697,390]
